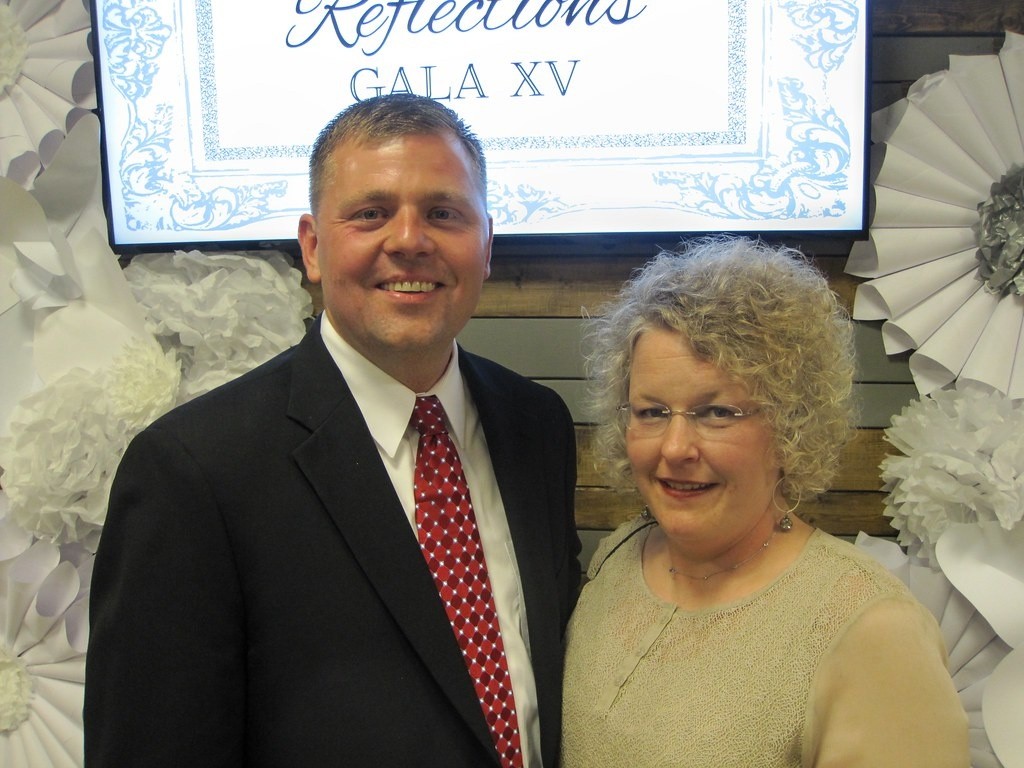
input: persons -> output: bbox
[82,95,581,768]
[562,239,973,768]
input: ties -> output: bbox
[410,397,525,768]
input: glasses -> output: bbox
[617,399,766,439]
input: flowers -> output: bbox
[875,383,1024,573]
[0,247,316,557]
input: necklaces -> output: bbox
[668,528,778,580]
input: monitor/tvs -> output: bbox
[90,0,873,262]
